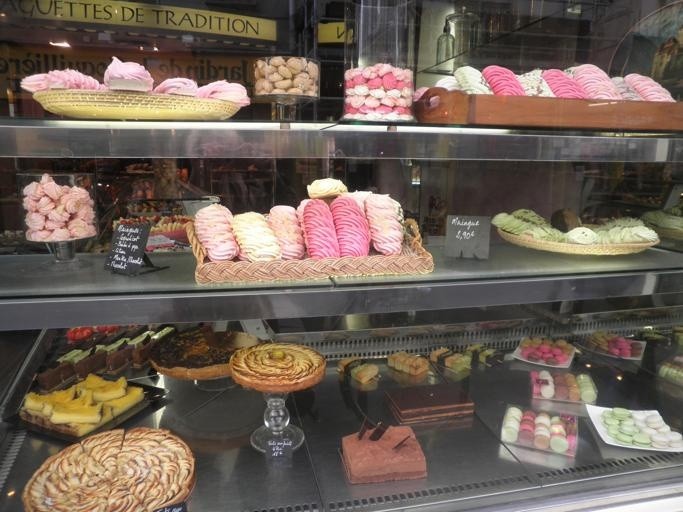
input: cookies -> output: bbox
[500,331,683,455]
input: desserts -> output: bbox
[22,172,683,263]
[20,325,501,512]
[21,55,683,126]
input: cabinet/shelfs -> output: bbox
[0,116,683,512]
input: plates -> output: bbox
[496,223,661,256]
[511,345,577,369]
[573,339,647,361]
[2,374,172,442]
[31,88,242,122]
[584,403,683,453]
